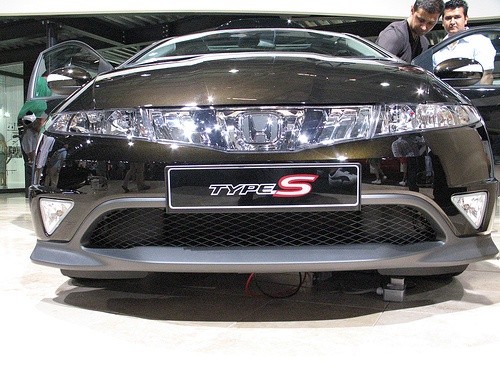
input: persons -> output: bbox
[366,134,433,192]
[433,0,496,86]
[0,133,8,186]
[375,0,446,73]
[17,70,152,193]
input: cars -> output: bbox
[18,26,499,297]
